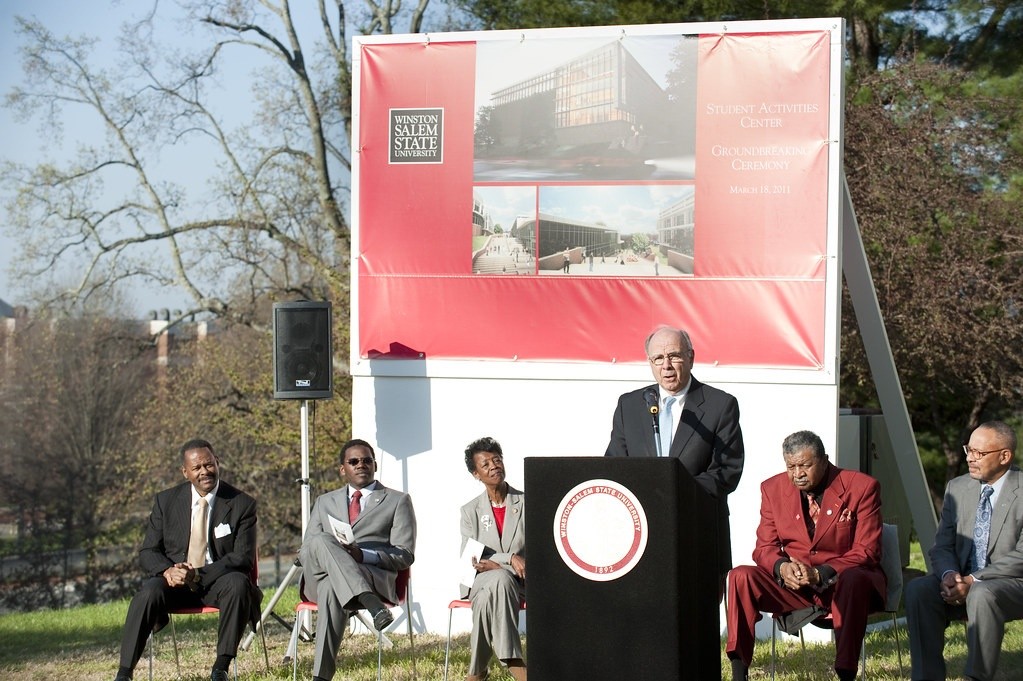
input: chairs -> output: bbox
[444,588,526,681]
[149,543,269,681]
[770,523,905,681]
[293,566,417,681]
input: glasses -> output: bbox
[344,457,375,466]
[963,445,1008,459]
[648,350,691,365]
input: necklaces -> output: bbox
[489,496,503,508]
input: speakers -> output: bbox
[272,300,333,400]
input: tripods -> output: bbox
[239,400,395,666]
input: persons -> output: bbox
[904,422,1023,681]
[477,245,530,275]
[296,439,418,681]
[726,431,889,681]
[604,324,745,571]
[459,437,527,681]
[115,439,264,681]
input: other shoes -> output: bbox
[466,668,487,681]
[508,657,527,681]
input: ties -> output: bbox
[806,492,821,541]
[970,485,994,573]
[186,498,209,592]
[349,491,362,525]
[659,397,676,457]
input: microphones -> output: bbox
[643,386,659,413]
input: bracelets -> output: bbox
[812,568,818,574]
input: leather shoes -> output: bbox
[374,607,394,631]
[211,668,228,681]
[114,675,132,681]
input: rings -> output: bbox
[956,600,961,604]
[347,549,351,553]
